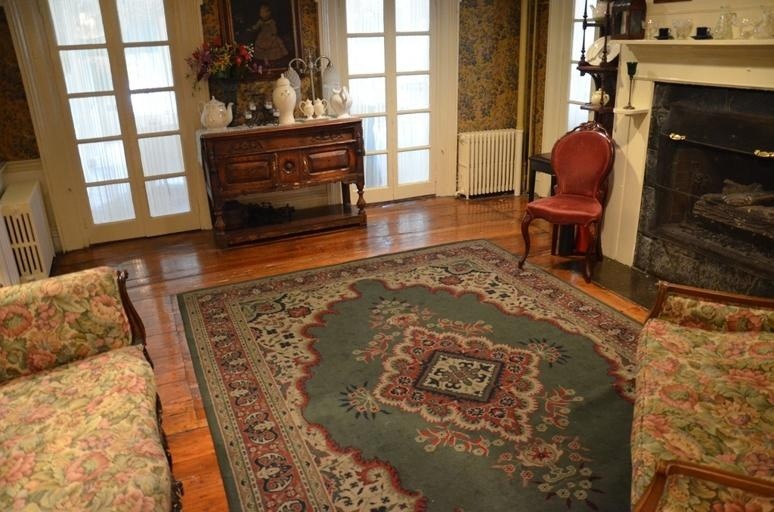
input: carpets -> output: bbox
[176,237,645,512]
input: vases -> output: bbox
[207,76,239,127]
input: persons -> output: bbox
[243,3,290,67]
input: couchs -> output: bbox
[629,279,773,512]
[0,265,183,512]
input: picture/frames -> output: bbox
[225,0,304,76]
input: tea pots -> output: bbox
[298,98,327,121]
[196,95,233,132]
[640,18,658,41]
[589,1,610,24]
[710,6,738,41]
[589,86,609,109]
[329,87,353,120]
[754,5,774,38]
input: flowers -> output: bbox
[183,36,263,96]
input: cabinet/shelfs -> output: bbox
[576,0,646,123]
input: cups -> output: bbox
[697,27,710,41]
[675,14,692,43]
[739,17,754,41]
[656,26,670,35]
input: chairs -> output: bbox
[515,121,616,284]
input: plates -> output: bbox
[583,34,624,66]
[653,35,673,41]
[691,34,714,41]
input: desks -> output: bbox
[201,117,367,250]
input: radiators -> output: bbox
[453,127,516,202]
[0,179,56,287]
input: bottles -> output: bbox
[271,74,298,128]
